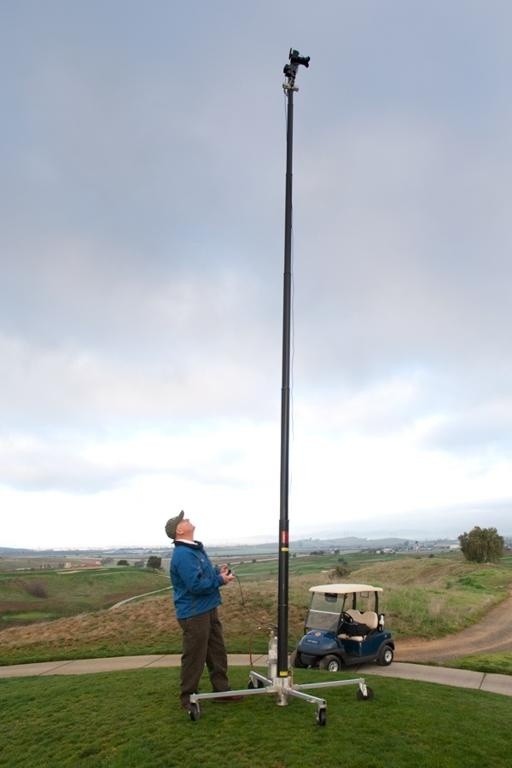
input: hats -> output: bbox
[165,510,185,539]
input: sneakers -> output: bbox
[212,695,243,703]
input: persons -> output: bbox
[166,509,245,704]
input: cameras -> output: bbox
[287,48,310,68]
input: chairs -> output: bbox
[337,607,360,656]
[347,605,383,658]
